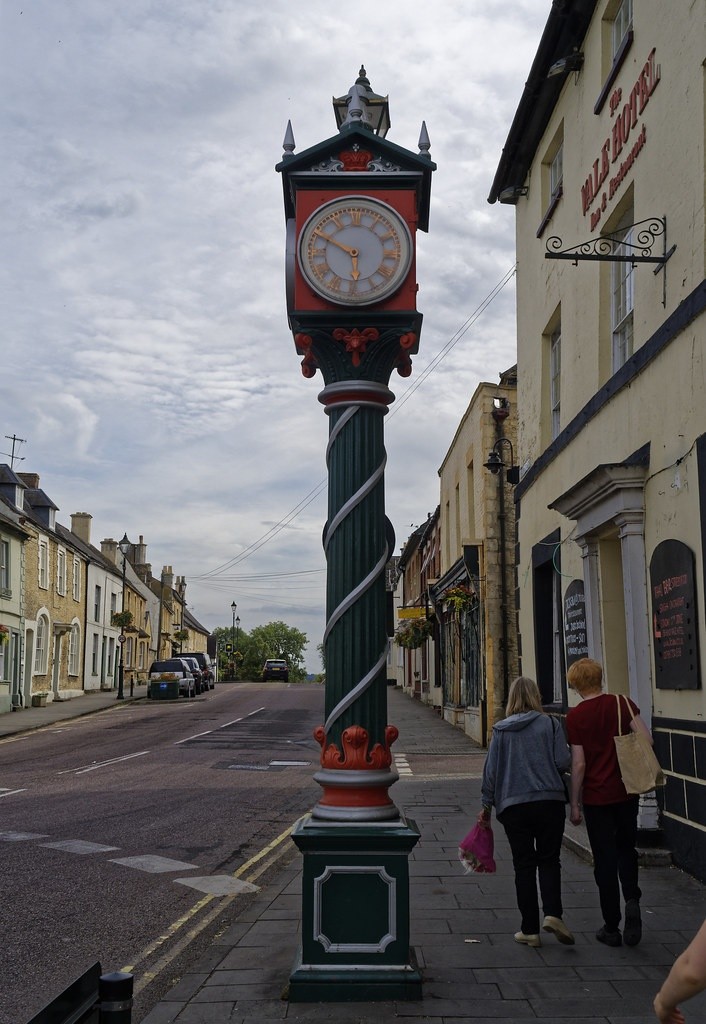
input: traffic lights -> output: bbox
[226,643,233,653]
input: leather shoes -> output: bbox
[542,915,576,944]
[514,931,541,946]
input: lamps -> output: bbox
[497,185,530,201]
[548,50,583,86]
[483,438,519,491]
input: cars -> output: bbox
[263,659,289,683]
[174,657,204,695]
[147,658,197,698]
[176,652,217,691]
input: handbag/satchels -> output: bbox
[548,714,572,804]
[614,694,667,794]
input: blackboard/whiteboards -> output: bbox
[649,539,703,691]
[563,579,588,689]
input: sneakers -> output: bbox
[596,924,622,945]
[623,898,642,945]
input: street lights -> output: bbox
[117,532,131,699]
[179,579,187,653]
[231,601,237,678]
[235,615,240,675]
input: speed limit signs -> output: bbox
[118,635,126,642]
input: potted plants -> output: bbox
[392,620,434,649]
[444,588,473,622]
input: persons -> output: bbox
[477,676,575,947]
[653,920,706,1024]
[564,658,654,947]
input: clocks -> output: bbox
[297,196,413,306]
